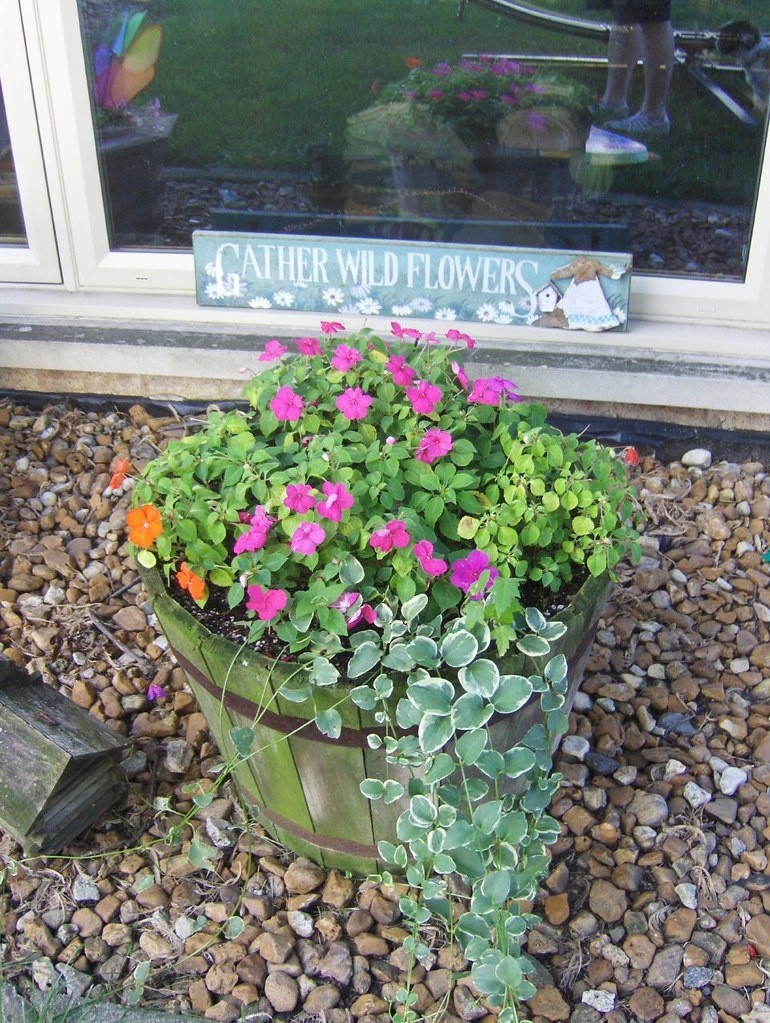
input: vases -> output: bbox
[137,566,610,877]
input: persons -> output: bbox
[586,0,672,135]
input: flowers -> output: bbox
[105,322,649,1023]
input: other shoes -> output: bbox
[537,125,648,166]
[603,111,671,136]
[586,98,629,121]
